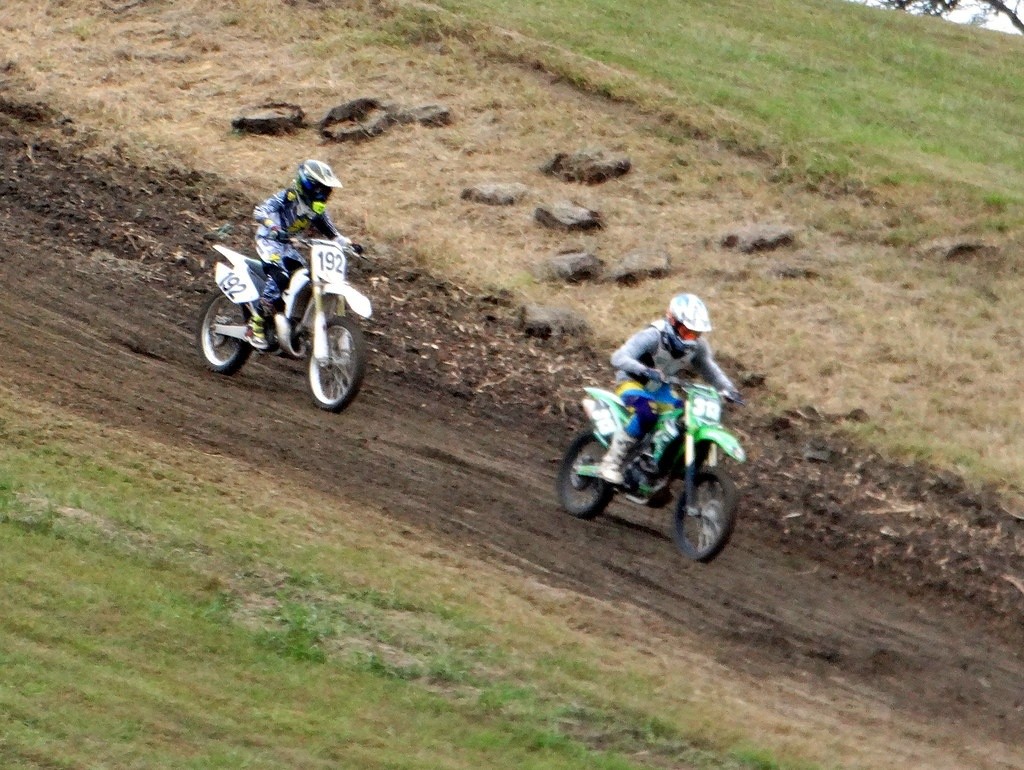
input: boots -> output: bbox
[598,429,637,484]
[244,309,268,350]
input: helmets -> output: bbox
[294,159,344,215]
[667,294,712,356]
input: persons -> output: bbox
[598,294,740,485]
[245,160,364,349]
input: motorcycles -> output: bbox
[194,229,373,416]
[552,369,747,566]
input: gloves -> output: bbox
[645,365,665,384]
[349,244,365,256]
[722,382,742,403]
[275,228,290,244]
